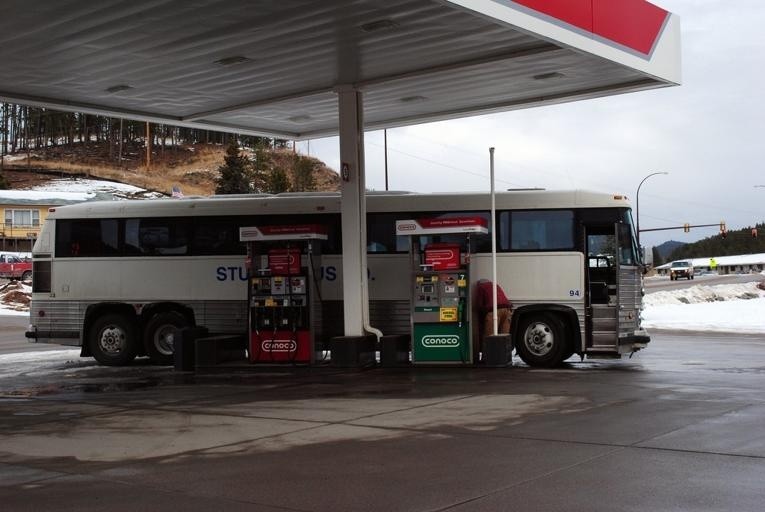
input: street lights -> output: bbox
[637,172,668,243]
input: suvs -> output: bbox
[670,260,694,280]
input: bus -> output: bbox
[26,189,649,366]
[0,251,32,280]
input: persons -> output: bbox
[474,279,513,366]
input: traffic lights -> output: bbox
[752,228,758,241]
